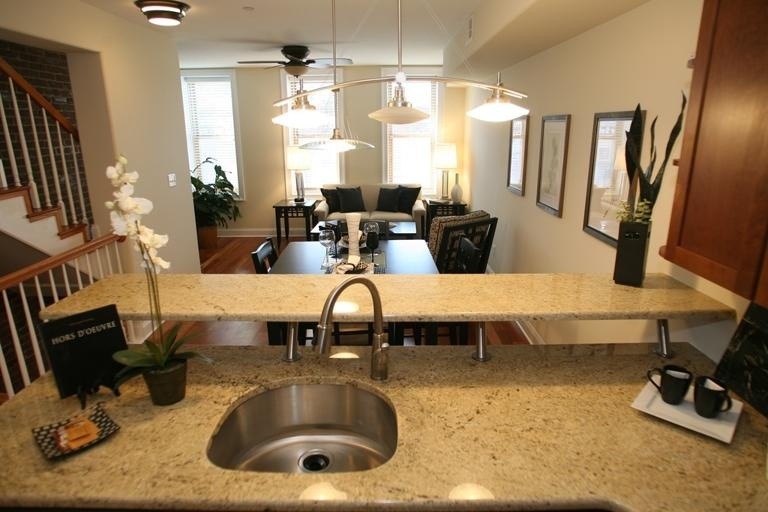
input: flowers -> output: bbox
[102,155,198,390]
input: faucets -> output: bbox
[315,276,389,381]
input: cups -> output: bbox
[693,375,734,420]
[645,361,694,405]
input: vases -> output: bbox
[141,359,187,406]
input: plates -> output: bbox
[30,403,121,460]
[629,373,744,444]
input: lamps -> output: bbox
[286,146,312,202]
[133,0,192,27]
[430,142,457,200]
[267,1,531,154]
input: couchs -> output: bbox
[311,183,426,239]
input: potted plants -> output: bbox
[190,156,242,249]
[612,89,687,288]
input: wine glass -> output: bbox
[325,215,349,259]
[364,221,380,257]
[320,230,336,268]
[366,232,379,268]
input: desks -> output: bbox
[272,198,318,248]
[309,220,417,240]
[421,199,467,242]
[38,269,735,322]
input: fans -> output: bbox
[237,44,353,78]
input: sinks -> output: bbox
[204,376,399,477]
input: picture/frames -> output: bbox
[581,108,647,250]
[534,112,571,219]
[505,115,529,198]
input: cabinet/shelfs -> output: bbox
[656,0,766,309]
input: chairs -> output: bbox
[250,236,318,346]
[426,209,497,273]
[394,236,481,345]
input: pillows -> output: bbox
[335,186,366,213]
[397,184,421,215]
[375,187,400,212]
[319,187,340,214]
